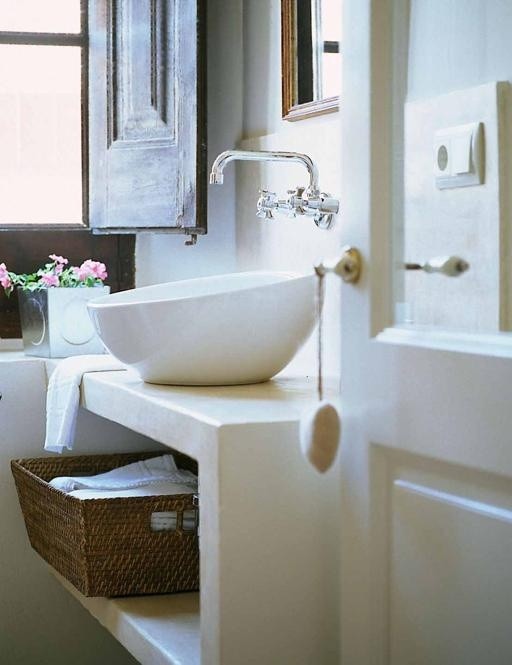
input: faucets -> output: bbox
[209,147,316,194]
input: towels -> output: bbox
[44,358,129,452]
[49,455,199,531]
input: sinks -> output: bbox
[86,269,322,386]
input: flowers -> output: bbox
[1,251,112,297]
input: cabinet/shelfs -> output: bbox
[36,363,346,665]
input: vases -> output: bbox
[15,285,113,357]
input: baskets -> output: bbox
[10,449,202,599]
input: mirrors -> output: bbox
[276,0,340,123]
